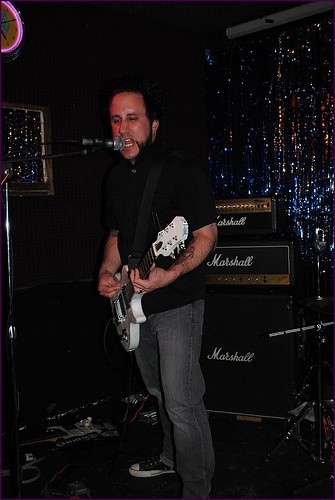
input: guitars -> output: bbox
[110,214,189,352]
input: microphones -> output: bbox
[70,138,124,151]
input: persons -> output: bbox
[98,75,219,500]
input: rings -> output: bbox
[139,288,143,294]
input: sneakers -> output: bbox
[128,457,177,477]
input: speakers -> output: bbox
[196,296,302,421]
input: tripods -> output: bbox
[264,239,334,465]
